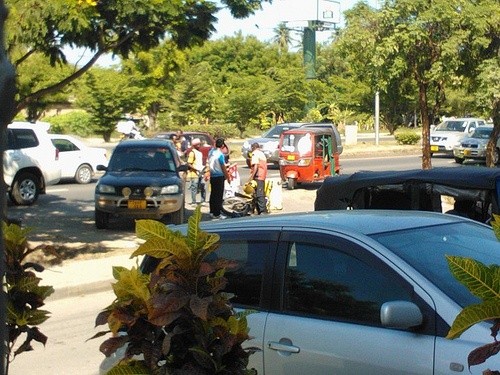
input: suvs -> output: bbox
[426,119,487,158]
[1,122,62,202]
[241,123,343,169]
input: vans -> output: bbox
[149,135,231,165]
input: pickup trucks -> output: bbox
[94,140,190,229]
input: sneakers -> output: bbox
[210,213,227,219]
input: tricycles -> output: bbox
[278,123,340,191]
[313,168,500,225]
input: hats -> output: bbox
[192,138,201,146]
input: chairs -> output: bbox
[296,249,338,283]
[154,152,169,169]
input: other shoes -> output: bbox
[191,201,208,206]
[260,212,267,215]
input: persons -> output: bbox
[209,139,232,216]
[170,134,228,205]
[187,138,209,205]
[250,143,268,214]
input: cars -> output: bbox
[454,127,500,163]
[144,210,500,375]
[52,134,105,184]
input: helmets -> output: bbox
[243,180,258,194]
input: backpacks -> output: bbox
[180,150,195,165]
